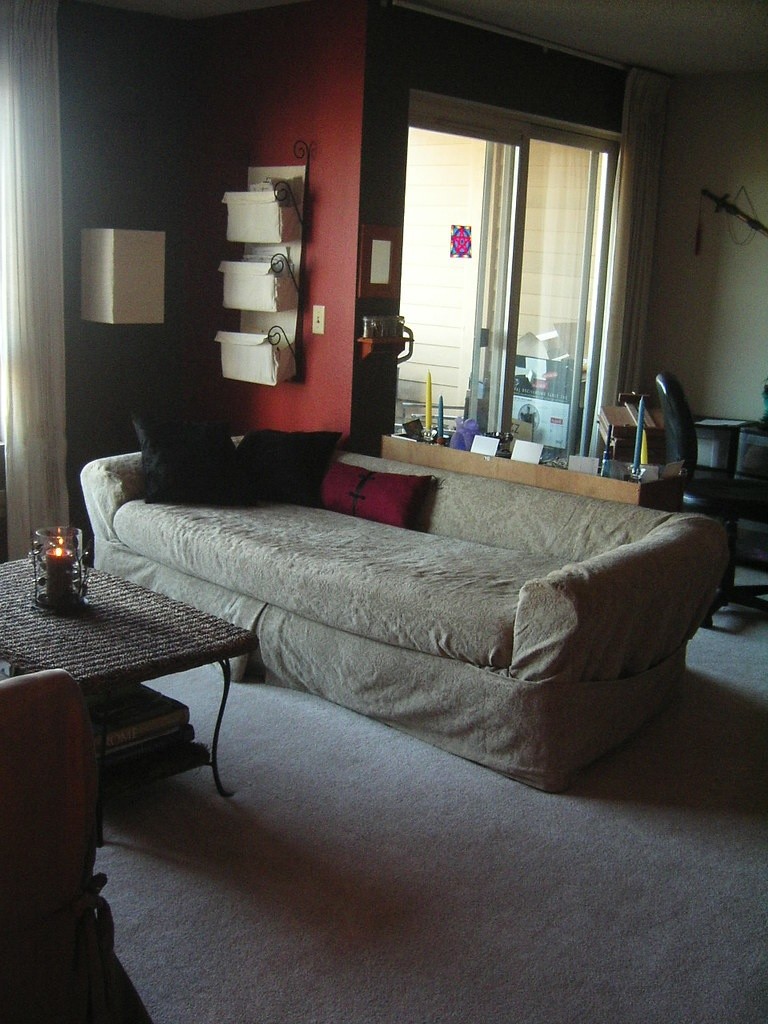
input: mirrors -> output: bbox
[358,223,403,300]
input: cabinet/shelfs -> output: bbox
[213,139,309,386]
[79,227,166,324]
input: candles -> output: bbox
[32,527,90,612]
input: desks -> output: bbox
[690,415,767,569]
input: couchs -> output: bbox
[80,435,729,792]
[0,668,121,1024]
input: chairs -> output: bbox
[656,371,768,631]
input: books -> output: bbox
[91,689,189,747]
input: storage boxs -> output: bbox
[512,329,577,449]
[383,430,689,509]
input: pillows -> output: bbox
[234,429,342,510]
[317,459,434,529]
[131,410,256,507]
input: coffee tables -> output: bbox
[0,555,258,846]
